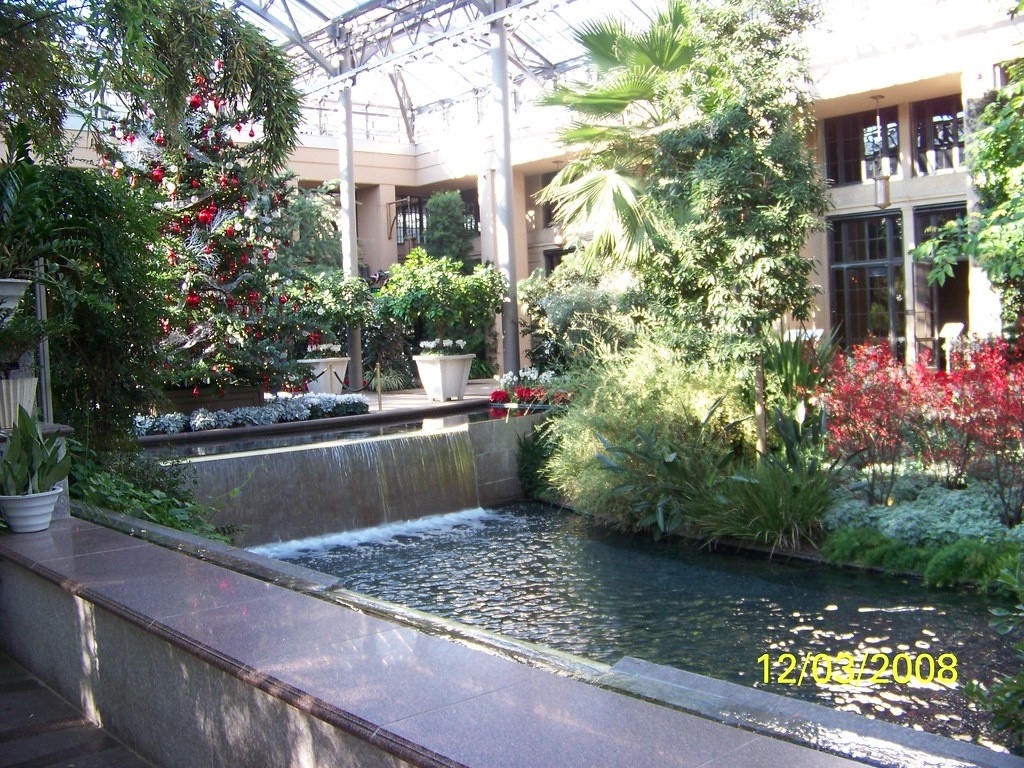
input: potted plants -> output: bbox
[0,301,78,428]
[0,403,72,533]
[0,122,106,331]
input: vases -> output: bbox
[297,357,351,395]
[411,353,476,402]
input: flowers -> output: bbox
[418,302,470,355]
[304,327,345,359]
